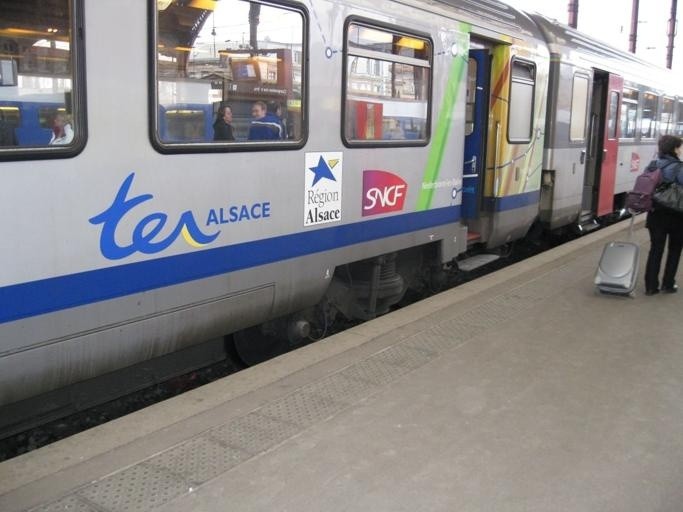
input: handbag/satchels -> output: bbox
[655,177,682,211]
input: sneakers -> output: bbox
[645,285,678,296]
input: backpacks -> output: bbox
[625,160,671,212]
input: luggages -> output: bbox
[594,240,641,297]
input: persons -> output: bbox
[639,134,682,297]
[211,106,236,142]
[46,112,74,146]
[244,100,284,141]
[271,103,284,120]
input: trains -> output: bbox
[0,3,683,453]
